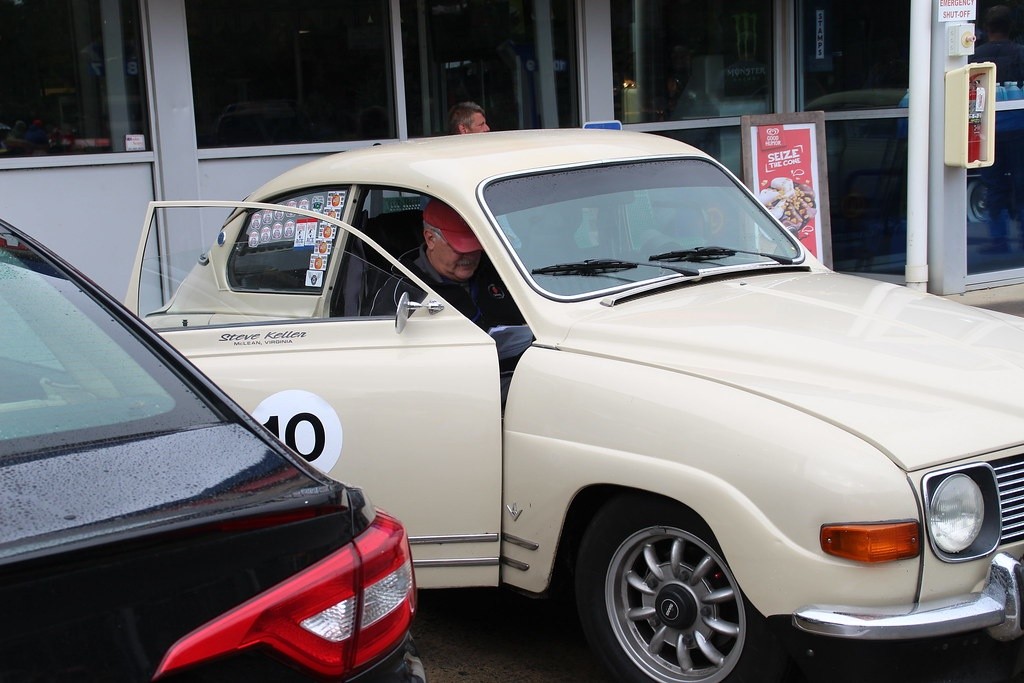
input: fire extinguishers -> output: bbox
[968,71,988,163]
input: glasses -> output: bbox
[430,231,484,258]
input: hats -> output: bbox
[423,196,483,253]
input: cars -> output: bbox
[123,128,1024,683]
[0,216,423,683]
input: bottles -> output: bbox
[896,88,910,134]
[995,81,1024,132]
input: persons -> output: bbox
[447,102,490,135]
[967,5,1024,255]
[363,198,536,417]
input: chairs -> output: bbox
[362,210,428,312]
